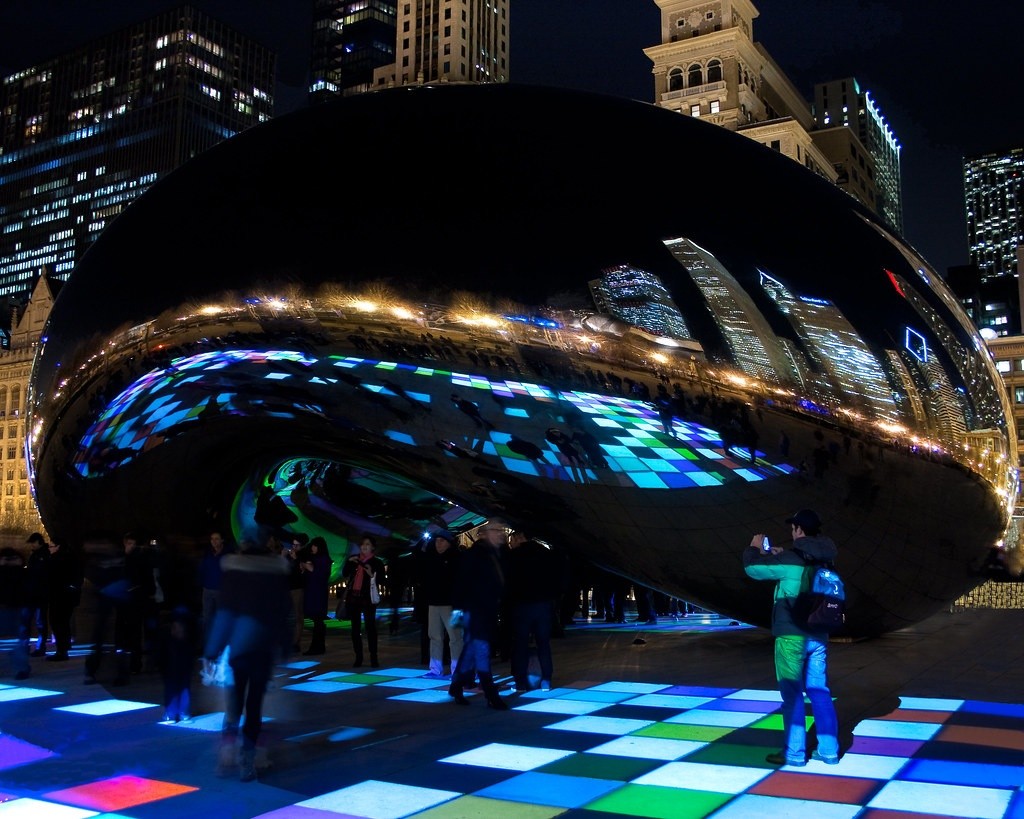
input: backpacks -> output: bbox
[786,547,847,639]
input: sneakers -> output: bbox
[766,748,839,767]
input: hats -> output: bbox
[432,530,451,542]
[27,533,42,542]
[785,508,823,530]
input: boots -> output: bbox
[218,739,257,781]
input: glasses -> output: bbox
[488,527,505,531]
[292,543,300,546]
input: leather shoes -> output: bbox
[484,690,508,710]
[449,682,472,704]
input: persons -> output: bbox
[201,545,293,782]
[52,316,877,517]
[412,519,702,712]
[743,511,840,766]
[344,537,384,669]
[1,532,332,725]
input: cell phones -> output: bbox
[763,536,771,553]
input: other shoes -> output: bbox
[45,654,69,662]
[631,617,656,625]
[515,676,551,690]
[353,658,380,668]
[164,712,193,727]
[31,649,45,657]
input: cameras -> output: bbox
[287,549,293,554]
[356,559,368,569]
